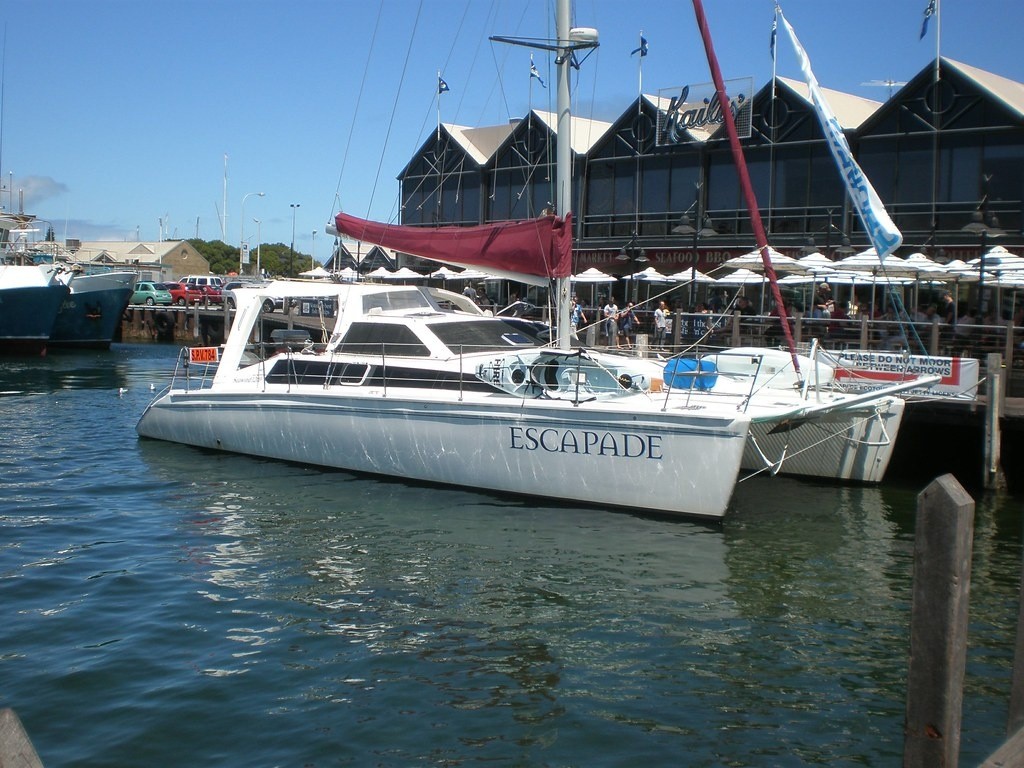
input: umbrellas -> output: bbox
[621,265,676,315]
[569,267,617,314]
[720,244,836,344]
[663,266,718,284]
[297,262,550,304]
[823,241,1024,324]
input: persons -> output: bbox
[840,290,1024,353]
[568,296,640,350]
[649,302,667,351]
[569,294,666,351]
[690,283,848,345]
[458,279,522,310]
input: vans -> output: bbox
[177,275,224,290]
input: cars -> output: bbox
[187,284,222,306]
[161,282,202,306]
[129,280,173,306]
[220,281,295,313]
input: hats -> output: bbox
[819,283,832,293]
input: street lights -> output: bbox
[239,191,266,276]
[311,230,317,258]
[290,204,301,244]
[253,217,262,275]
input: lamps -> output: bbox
[961,209,1008,238]
[614,232,650,262]
[671,200,719,237]
[800,224,857,253]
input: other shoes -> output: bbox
[660,347,664,350]
[648,343,651,350]
[616,346,622,349]
[629,347,633,350]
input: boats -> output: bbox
[0,171,138,349]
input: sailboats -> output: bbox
[134,1,943,522]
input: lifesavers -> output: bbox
[206,316,225,338]
[155,311,176,335]
